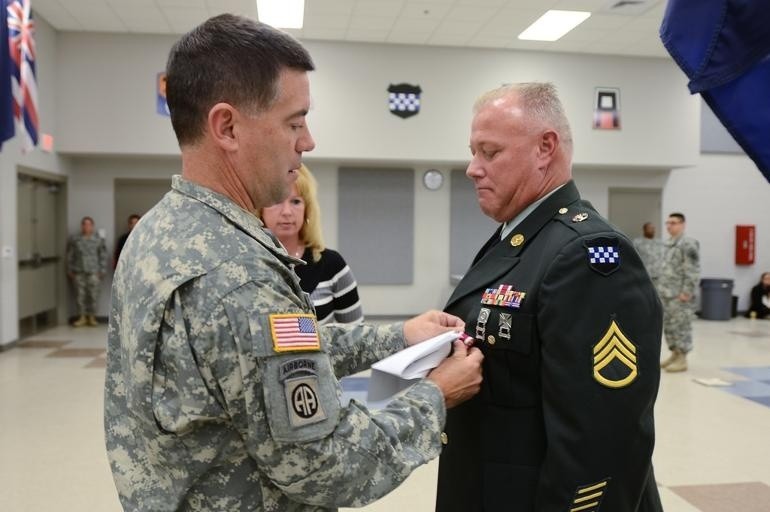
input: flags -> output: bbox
[5,0,45,149]
[0,1,16,151]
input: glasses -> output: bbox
[664,219,678,225]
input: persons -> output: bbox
[631,221,664,286]
[649,212,703,373]
[419,76,669,512]
[743,271,770,322]
[62,216,111,327]
[245,156,369,328]
[105,13,485,512]
[113,215,144,266]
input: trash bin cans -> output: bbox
[700,279,734,321]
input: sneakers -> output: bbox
[73,314,100,328]
[659,348,689,373]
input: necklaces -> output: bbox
[289,242,302,271]
